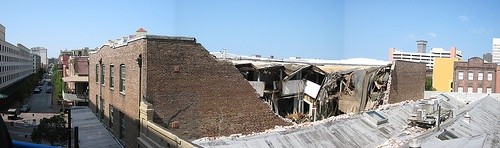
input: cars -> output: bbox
[7,108,17,119]
[38,86,42,91]
[20,104,31,113]
[33,87,41,94]
[38,63,54,87]
[45,88,52,93]
